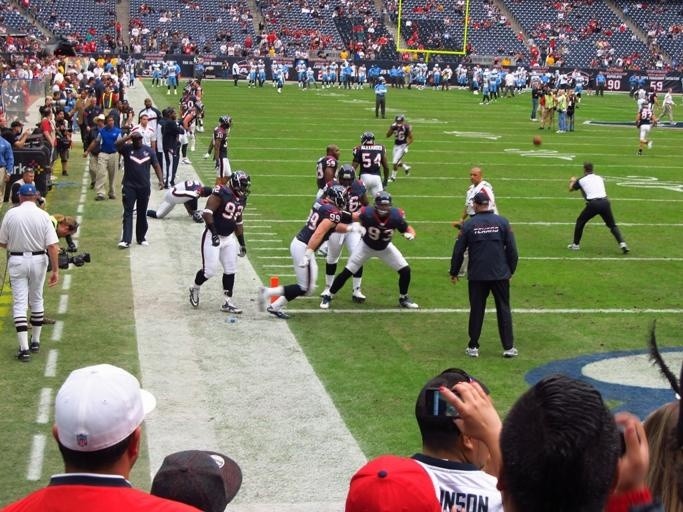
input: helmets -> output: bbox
[338,164,356,186]
[219,115,232,130]
[229,170,251,201]
[395,115,405,126]
[374,191,392,218]
[360,132,375,145]
[326,185,347,210]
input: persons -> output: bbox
[40,109,58,181]
[100,2,221,53]
[580,17,630,54]
[496,375,655,512]
[294,60,366,90]
[623,3,683,54]
[0,183,59,362]
[84,113,106,191]
[404,0,476,53]
[516,1,579,53]
[386,116,414,183]
[636,73,645,89]
[113,132,165,249]
[8,170,47,209]
[634,86,646,109]
[318,165,368,300]
[139,97,162,127]
[467,4,513,33]
[217,2,334,54]
[163,108,185,187]
[316,144,340,199]
[2,1,98,53]
[451,191,521,359]
[189,170,251,313]
[0,129,15,212]
[334,0,397,52]
[646,87,660,110]
[232,59,289,94]
[566,161,631,253]
[352,133,389,200]
[51,110,73,176]
[629,73,638,95]
[83,114,122,201]
[203,116,233,178]
[0,364,203,511]
[411,367,496,512]
[0,121,31,150]
[367,58,453,92]
[657,88,677,125]
[596,71,607,97]
[634,386,683,510]
[375,77,388,119]
[455,55,586,134]
[446,167,499,278]
[10,214,79,328]
[322,191,419,310]
[344,378,507,512]
[256,186,367,319]
[128,114,156,151]
[635,102,657,156]
[123,177,212,220]
[590,55,672,72]
[1,54,207,136]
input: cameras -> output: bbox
[46,234,91,273]
[58,128,73,151]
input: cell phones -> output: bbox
[425,388,463,417]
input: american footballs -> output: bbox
[533,135,543,145]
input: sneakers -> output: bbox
[15,351,30,361]
[320,295,331,309]
[388,177,396,183]
[118,241,129,248]
[503,344,519,357]
[220,299,243,314]
[140,241,149,246]
[267,305,290,319]
[352,288,366,303]
[189,286,200,307]
[464,347,479,357]
[399,295,419,309]
[29,342,39,352]
[404,165,412,177]
[619,242,630,253]
[567,243,580,249]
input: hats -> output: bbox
[10,121,25,130]
[93,114,105,124]
[16,184,37,196]
[346,456,442,511]
[470,193,489,205]
[55,364,157,452]
